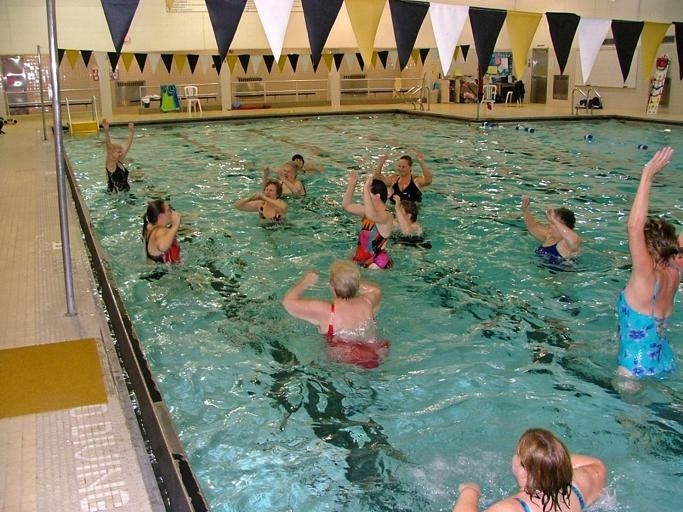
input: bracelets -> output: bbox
[561,226,567,236]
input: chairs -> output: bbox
[504,80,525,104]
[184,86,203,112]
[480,86,497,110]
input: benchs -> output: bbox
[235,89,316,97]
[8,99,93,115]
[341,88,411,94]
[142,94,219,107]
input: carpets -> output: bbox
[0,338,108,419]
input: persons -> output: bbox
[374,148,433,205]
[389,193,432,255]
[99,119,135,202]
[139,201,186,266]
[234,182,287,226]
[518,193,582,283]
[613,144,683,464]
[262,160,307,201]
[341,170,396,272]
[265,154,325,176]
[453,428,605,511]
[281,257,391,377]
